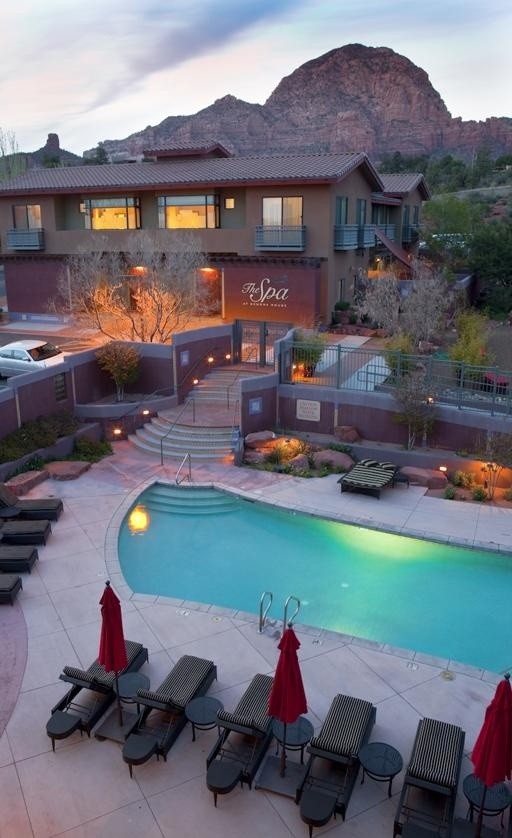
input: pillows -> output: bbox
[378,461,397,471]
[360,456,378,468]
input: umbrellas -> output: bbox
[96,578,130,727]
[468,673,511,838]
[263,621,309,778]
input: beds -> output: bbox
[336,459,399,500]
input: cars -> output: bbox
[0,339,77,378]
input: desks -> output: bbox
[464,771,512,838]
[392,474,410,489]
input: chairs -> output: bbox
[0,481,65,607]
